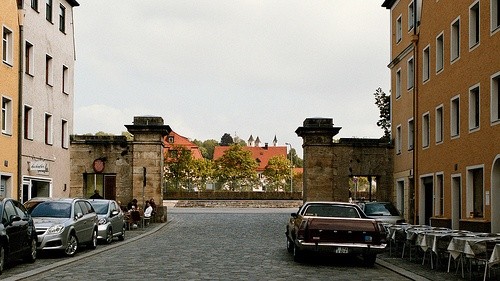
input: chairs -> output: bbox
[129,206,157,231]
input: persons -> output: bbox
[89,190,103,199]
[142,198,156,226]
[117,201,126,210]
[127,199,137,209]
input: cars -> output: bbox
[350,202,406,226]
[26,198,99,256]
[0,195,38,276]
[87,198,127,244]
[24,197,50,215]
[284,201,387,268]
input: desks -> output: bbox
[379,223,500,281]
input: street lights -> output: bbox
[284,142,293,198]
